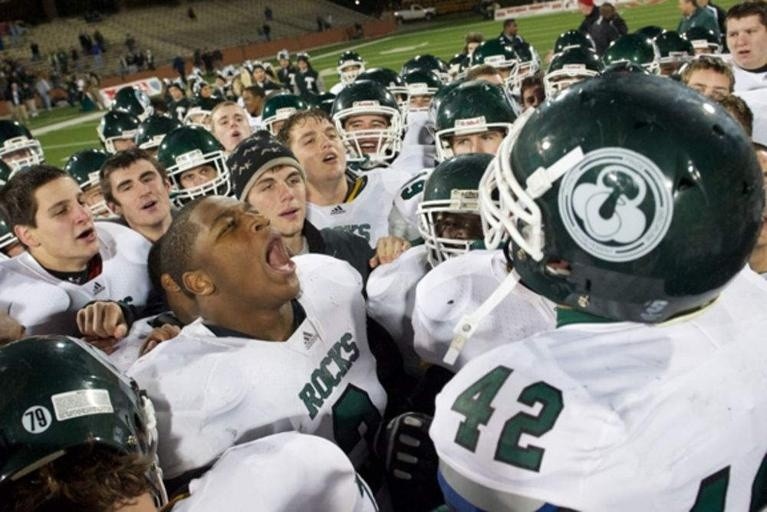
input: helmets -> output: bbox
[338,48,365,72]
[419,152,494,270]
[554,29,598,52]
[0,118,48,176]
[157,124,230,210]
[433,83,517,137]
[97,111,144,154]
[471,40,540,72]
[261,95,310,130]
[354,67,407,107]
[0,337,159,480]
[64,150,114,190]
[481,69,764,323]
[328,80,406,170]
[395,69,442,109]
[402,54,449,75]
[112,86,151,113]
[634,24,667,46]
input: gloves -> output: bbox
[368,411,444,512]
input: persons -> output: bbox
[0,121,46,259]
[366,151,560,411]
[674,2,721,60]
[522,76,545,110]
[680,58,736,109]
[679,25,722,59]
[124,194,438,512]
[632,23,666,59]
[90,147,184,246]
[124,31,134,50]
[94,51,319,147]
[493,17,536,65]
[699,0,730,51]
[330,82,427,246]
[316,11,336,34]
[744,136,767,283]
[279,110,394,242]
[0,34,94,119]
[263,20,272,39]
[264,4,271,20]
[103,233,198,376]
[449,32,486,68]
[395,81,522,218]
[712,94,754,153]
[93,28,106,48]
[429,69,767,511]
[354,21,364,37]
[328,48,368,96]
[721,0,767,149]
[475,34,523,68]
[402,51,441,90]
[187,6,196,20]
[227,129,411,415]
[553,30,594,54]
[574,1,625,65]
[0,334,379,512]
[2,165,153,350]
[597,1,630,36]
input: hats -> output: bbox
[227,129,306,200]
